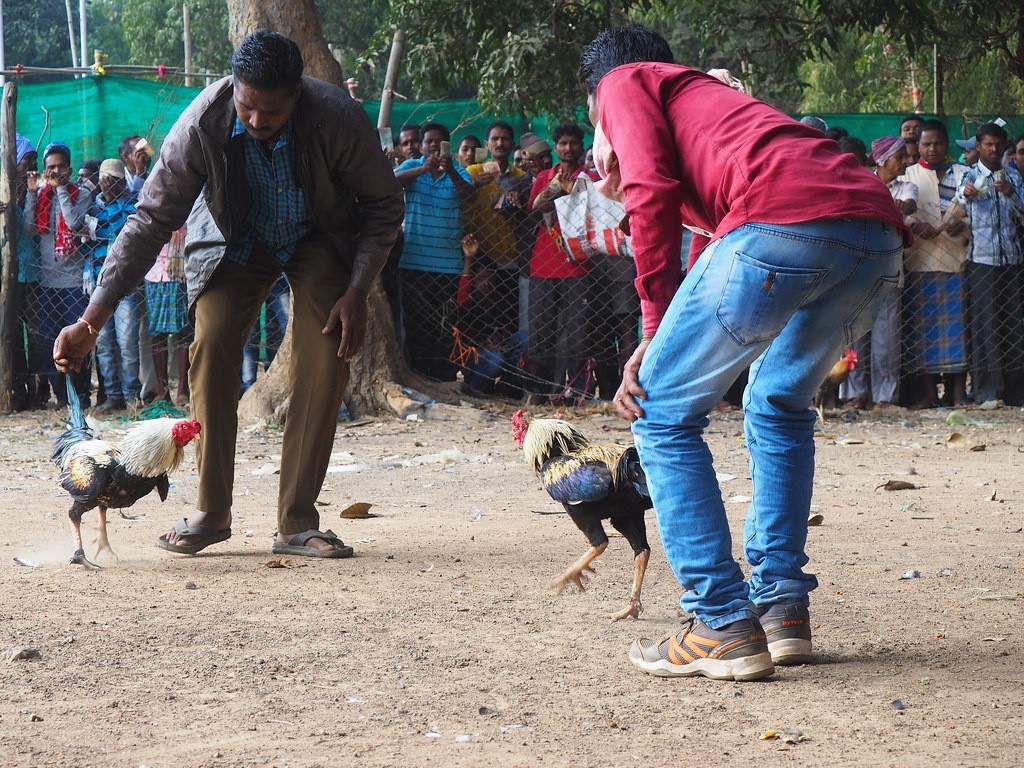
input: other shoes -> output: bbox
[10,385,188,416]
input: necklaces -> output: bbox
[266,134,279,150]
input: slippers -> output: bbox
[157,517,232,554]
[272,529,353,558]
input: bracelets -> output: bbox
[77,318,100,336]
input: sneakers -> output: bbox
[630,611,774,681]
[760,600,811,658]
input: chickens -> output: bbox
[512,408,657,622]
[810,348,859,440]
[52,371,203,570]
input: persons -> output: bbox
[12,132,292,410]
[579,26,916,681]
[384,122,753,406]
[52,31,407,558]
[803,114,1024,410]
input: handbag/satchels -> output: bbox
[554,171,635,261]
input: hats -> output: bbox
[521,132,551,159]
[955,135,976,149]
[15,133,39,166]
[78,161,101,178]
[98,159,127,181]
[43,141,69,162]
[872,137,905,165]
